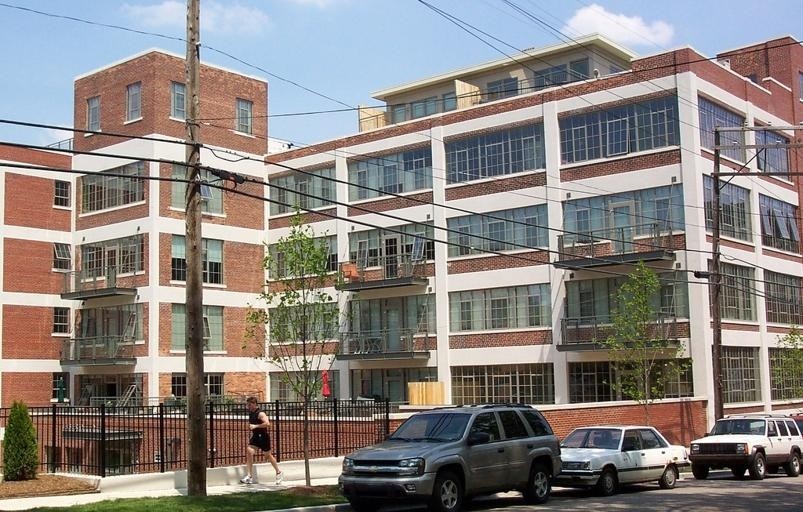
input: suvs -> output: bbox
[782,411,803,440]
[338,398,562,511]
[687,411,802,480]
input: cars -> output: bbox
[553,422,690,494]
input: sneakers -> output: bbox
[240,475,253,483]
[276,471,282,484]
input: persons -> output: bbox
[238,396,286,487]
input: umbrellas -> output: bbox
[320,367,331,400]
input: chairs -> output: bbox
[342,265,365,284]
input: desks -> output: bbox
[352,337,383,354]
